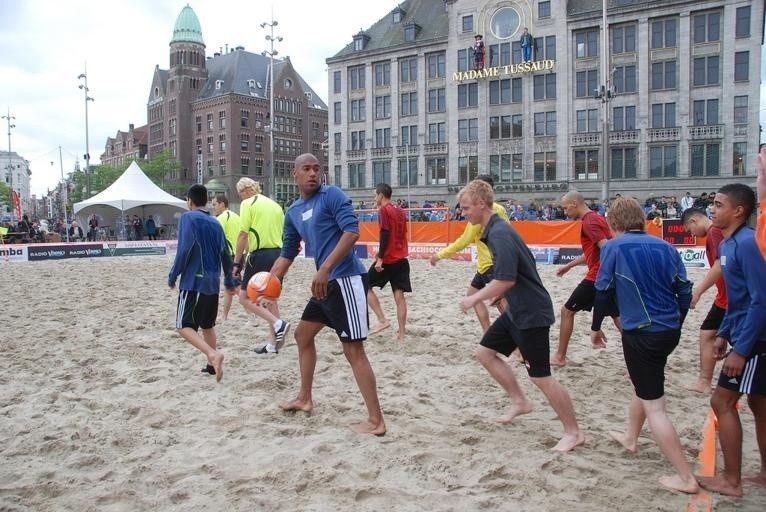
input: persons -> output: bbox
[456,179,585,451]
[472,35,484,70]
[248,153,386,435]
[591,197,700,494]
[357,199,465,221]
[5,213,98,243]
[125,214,157,240]
[681,208,728,395]
[232,177,291,354]
[211,196,241,322]
[549,190,622,367]
[642,192,716,220]
[430,175,510,333]
[692,183,766,497]
[505,194,639,222]
[167,184,233,383]
[520,27,534,63]
[363,183,412,337]
[755,148,766,262]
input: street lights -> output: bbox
[77,64,96,197]
[1,109,16,225]
[260,17,282,203]
[594,69,620,208]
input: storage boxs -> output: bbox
[108,236,117,241]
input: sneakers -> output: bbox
[274,320,290,350]
[253,345,277,354]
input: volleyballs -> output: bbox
[247,271,281,307]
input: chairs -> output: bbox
[0,217,86,244]
[356,205,539,222]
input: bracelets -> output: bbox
[233,263,241,268]
